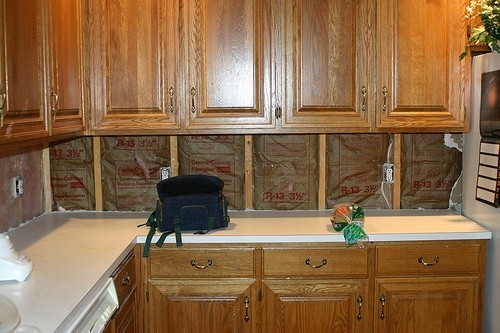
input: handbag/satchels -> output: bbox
[137,174,231,257]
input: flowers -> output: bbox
[458,0,500,60]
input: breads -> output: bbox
[332,204,364,223]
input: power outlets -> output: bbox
[382,164,394,183]
[159,167,171,181]
[12,176,24,197]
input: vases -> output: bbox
[469,45,491,58]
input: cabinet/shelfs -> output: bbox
[103,239,487,333]
[0,0,471,152]
